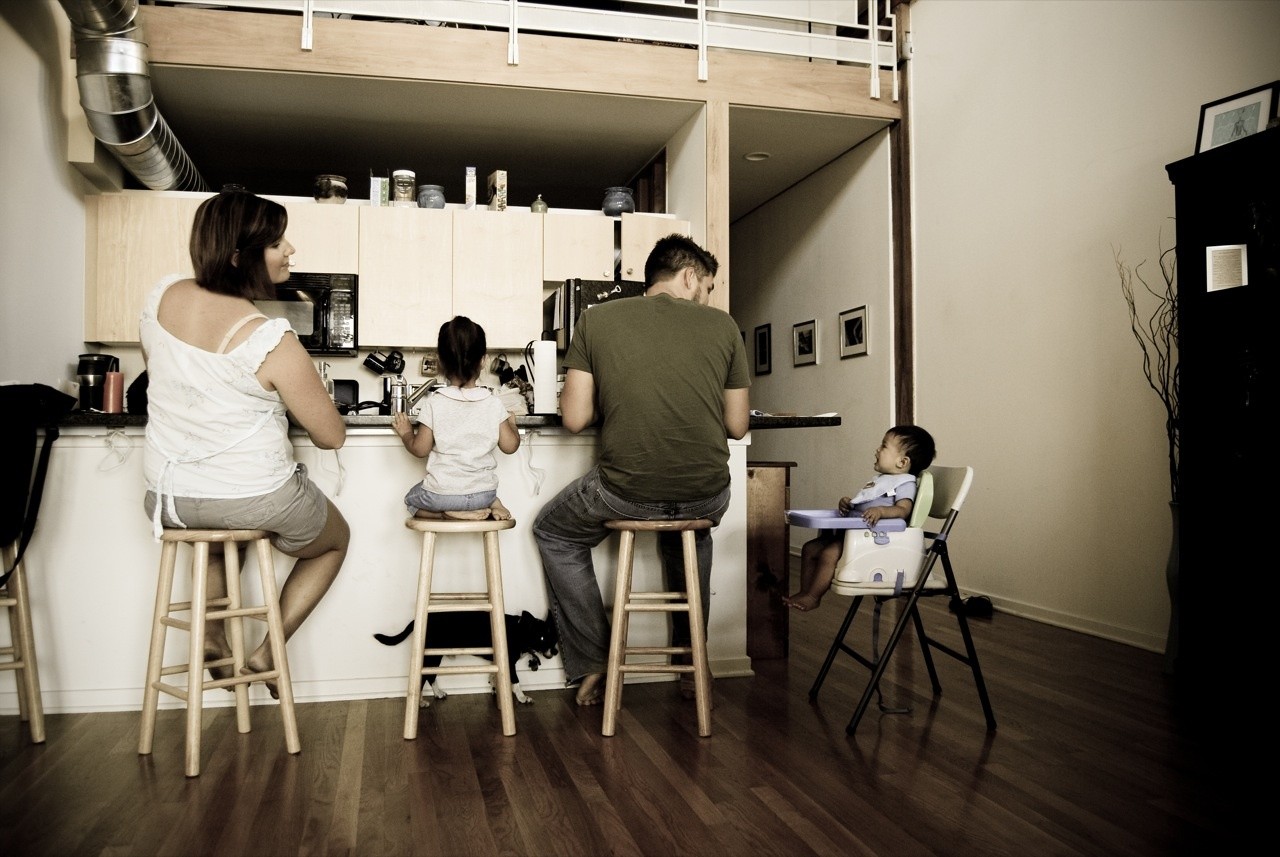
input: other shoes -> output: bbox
[948,595,993,621]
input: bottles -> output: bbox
[313,175,347,204]
[417,185,446,208]
[531,194,548,212]
[221,184,244,192]
[601,187,636,216]
[393,169,416,201]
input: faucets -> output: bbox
[390,378,437,415]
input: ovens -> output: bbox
[542,278,648,351]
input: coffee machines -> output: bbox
[75,353,120,415]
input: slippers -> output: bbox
[240,667,278,699]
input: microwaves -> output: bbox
[251,271,358,357]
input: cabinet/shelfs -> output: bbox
[85,189,692,346]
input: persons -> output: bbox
[139,187,350,700]
[530,233,752,708]
[781,424,937,614]
[391,316,520,522]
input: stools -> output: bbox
[0,536,46,742]
[136,526,299,776]
[597,520,716,739]
[402,517,515,741]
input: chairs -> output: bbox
[809,466,996,733]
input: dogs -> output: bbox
[373,608,559,707]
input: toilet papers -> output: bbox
[531,340,559,413]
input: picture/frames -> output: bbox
[754,323,772,376]
[838,305,868,360]
[792,319,817,369]
[1193,80,1280,154]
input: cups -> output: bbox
[103,371,124,414]
[491,352,510,374]
[385,351,406,374]
[421,352,441,375]
[362,350,388,374]
